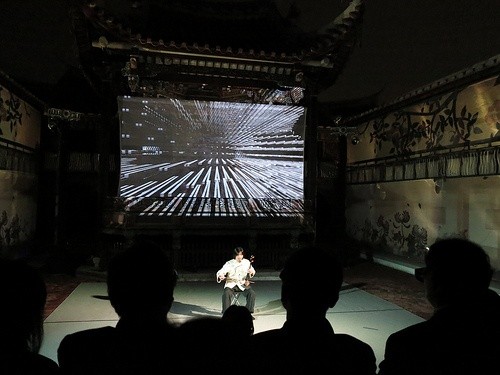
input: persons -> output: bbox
[216,247,255,313]
[0,252,500,375]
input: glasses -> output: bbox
[415,267,426,284]
